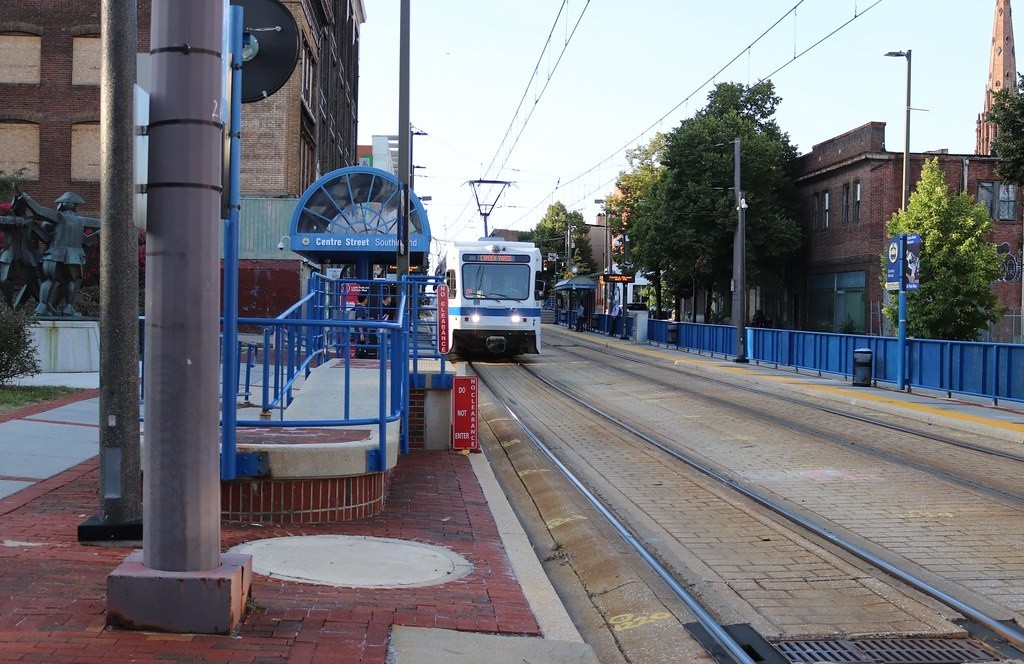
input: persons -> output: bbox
[354,291,367,359]
[608,300,619,337]
[382,293,396,321]
[574,302,584,332]
[12,180,100,315]
[0,194,56,313]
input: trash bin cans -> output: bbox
[852,348,873,386]
[591,313,599,329]
[667,323,678,343]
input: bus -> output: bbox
[436,236,545,360]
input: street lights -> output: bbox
[409,131,431,202]
[883,50,912,390]
[561,220,573,328]
[595,198,612,317]
